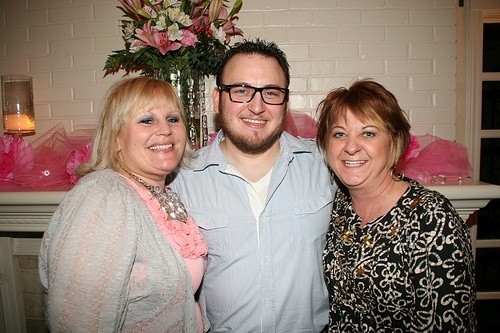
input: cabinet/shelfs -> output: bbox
[0,178,500,333]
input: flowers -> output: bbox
[102,0,244,78]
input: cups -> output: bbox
[3,75,38,137]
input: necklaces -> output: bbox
[119,164,188,223]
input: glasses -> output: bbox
[218,84,289,105]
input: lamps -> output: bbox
[1,74,35,136]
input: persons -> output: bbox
[166,35,342,333]
[38,76,209,333]
[312,79,478,333]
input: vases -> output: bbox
[157,78,208,151]
